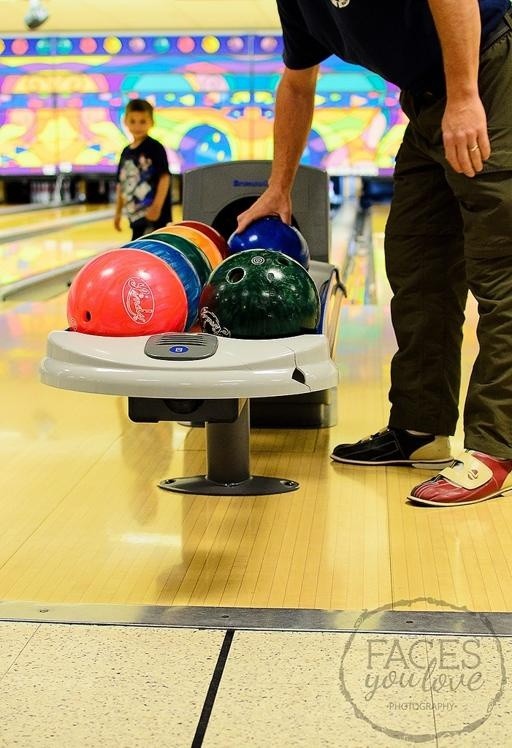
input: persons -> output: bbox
[114,99,172,241]
[236,0,512,505]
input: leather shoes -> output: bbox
[329,423,512,506]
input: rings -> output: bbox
[470,145,478,152]
